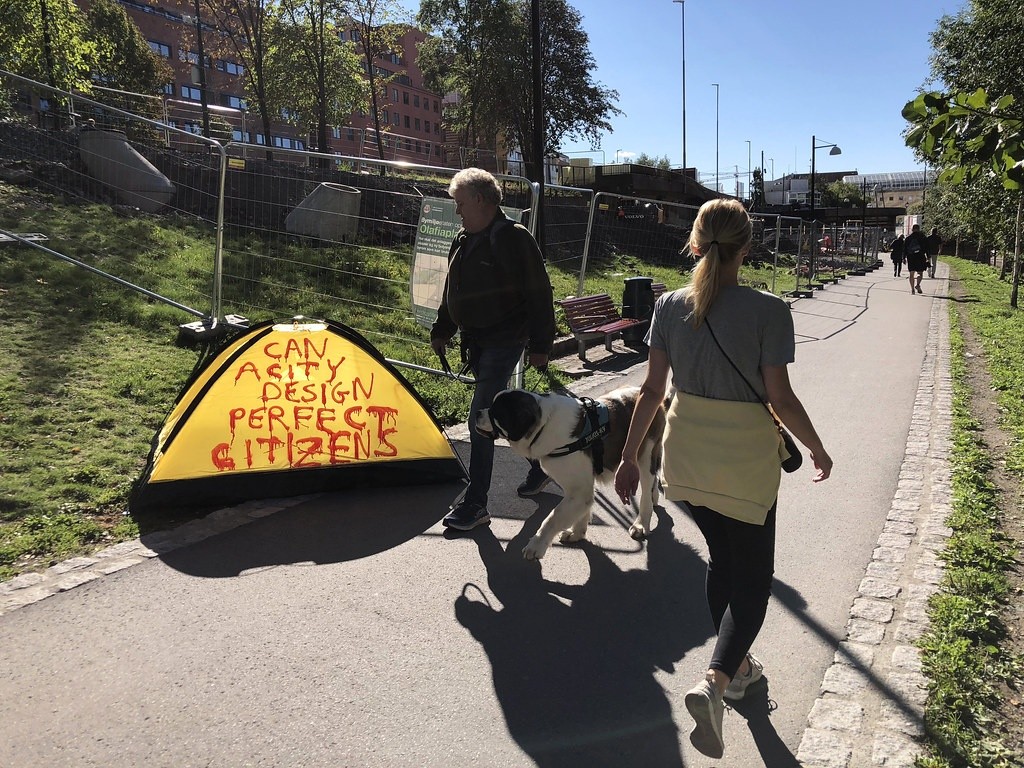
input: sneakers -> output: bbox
[517,467,552,495]
[442,502,491,530]
[723,652,764,700]
[684,669,724,759]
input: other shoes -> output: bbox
[911,291,915,294]
[915,284,922,294]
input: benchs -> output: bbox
[650,283,668,299]
[554,294,648,359]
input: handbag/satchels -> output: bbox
[777,426,802,473]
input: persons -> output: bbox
[81,118,98,131]
[613,198,834,760]
[926,227,942,279]
[889,233,905,277]
[429,166,558,531]
[902,224,931,295]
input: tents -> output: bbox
[127,315,471,517]
